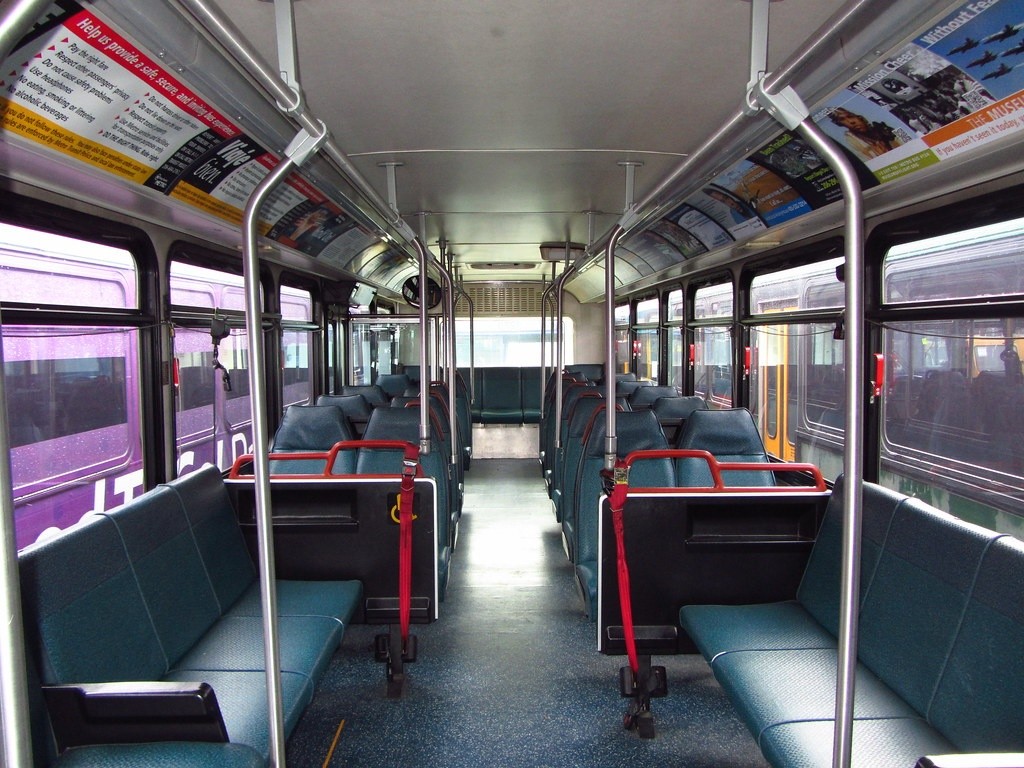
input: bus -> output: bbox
[624,228,1023,544]
[0,240,397,554]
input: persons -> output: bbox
[902,75,997,136]
[826,107,906,160]
[703,187,755,224]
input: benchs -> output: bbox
[18,369,474,768]
[541,363,1024,768]
[396,361,545,430]
